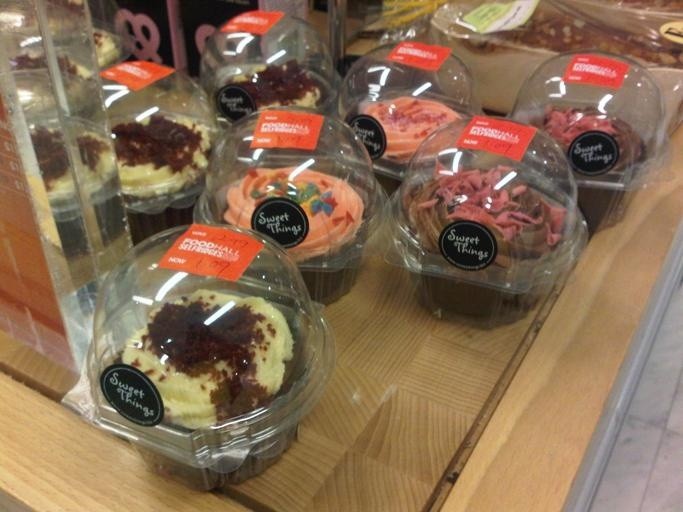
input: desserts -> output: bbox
[1,1,683,491]
